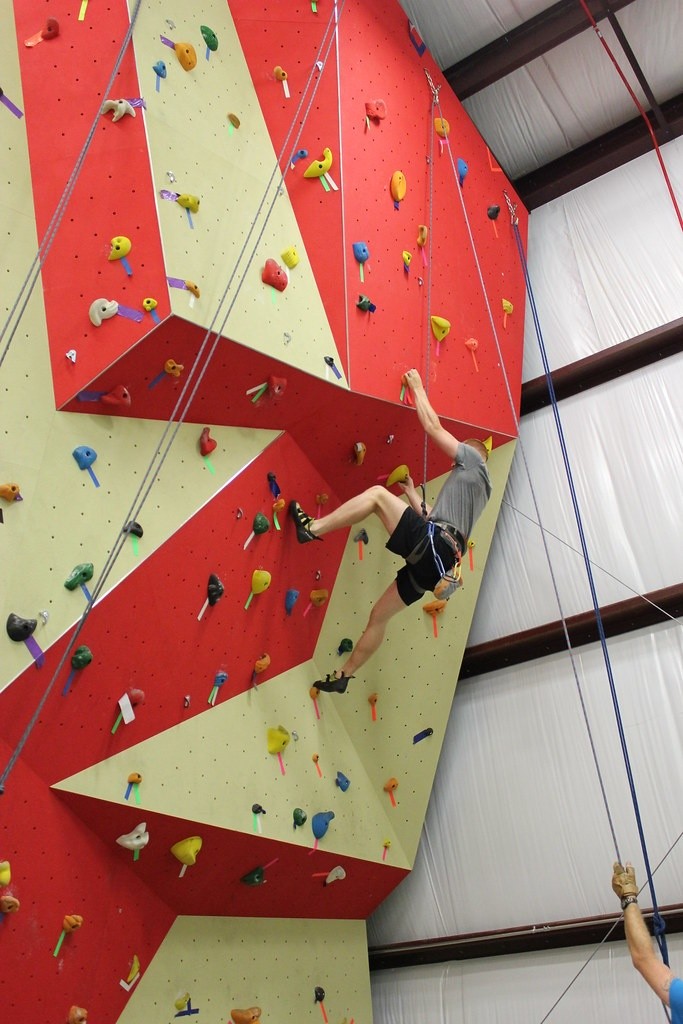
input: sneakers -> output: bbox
[312,670,351,694]
[290,502,316,543]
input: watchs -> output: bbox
[621,898,638,910]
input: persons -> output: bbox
[612,862,683,1024]
[289,367,492,695]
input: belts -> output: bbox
[433,522,466,557]
